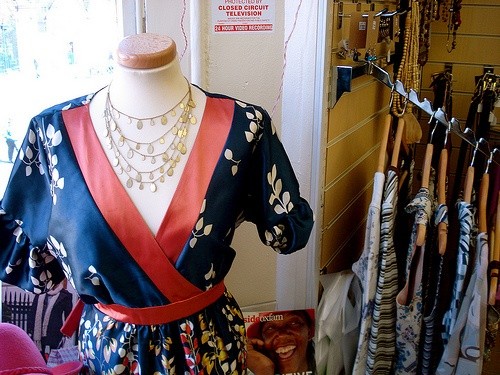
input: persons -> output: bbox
[26,278,75,358]
[243,309,317,375]
[0,33,314,375]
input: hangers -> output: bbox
[375,82,500,305]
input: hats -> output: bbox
[247,309,316,341]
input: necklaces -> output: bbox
[103,77,196,192]
